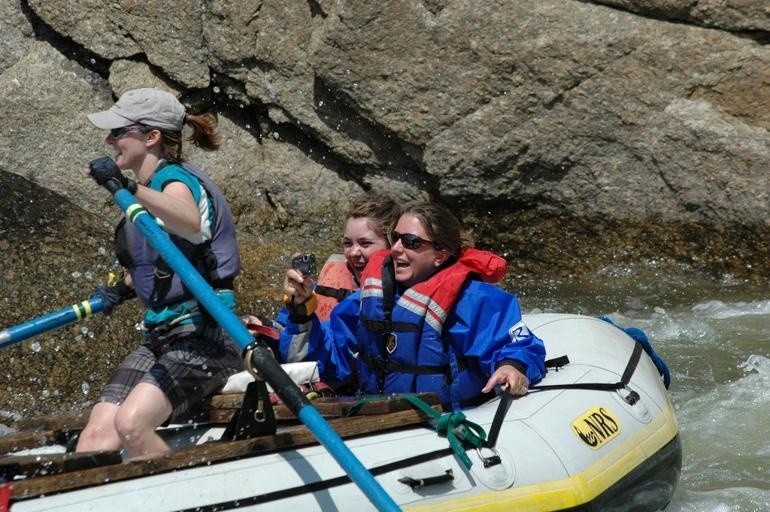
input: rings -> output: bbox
[522,383,528,393]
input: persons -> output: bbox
[241,191,395,368]
[277,204,548,414]
[73,88,243,460]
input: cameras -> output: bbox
[291,254,317,276]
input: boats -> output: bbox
[3,311,686,512]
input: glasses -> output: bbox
[111,124,145,139]
[390,230,435,251]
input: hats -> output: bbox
[85,87,187,132]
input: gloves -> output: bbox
[87,156,137,195]
[98,280,131,316]
